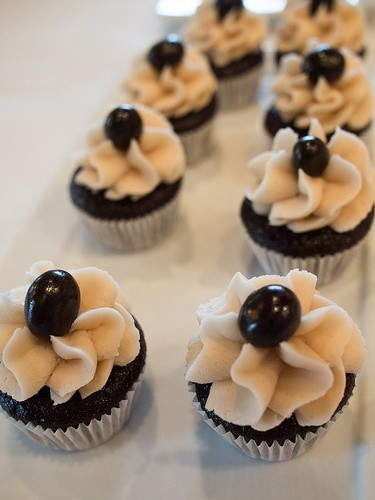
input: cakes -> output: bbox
[118,37,219,162]
[268,0,367,70]
[178,0,267,107]
[68,103,186,252]
[262,41,374,140]
[0,260,146,452]
[238,118,375,288]
[185,267,364,462]
